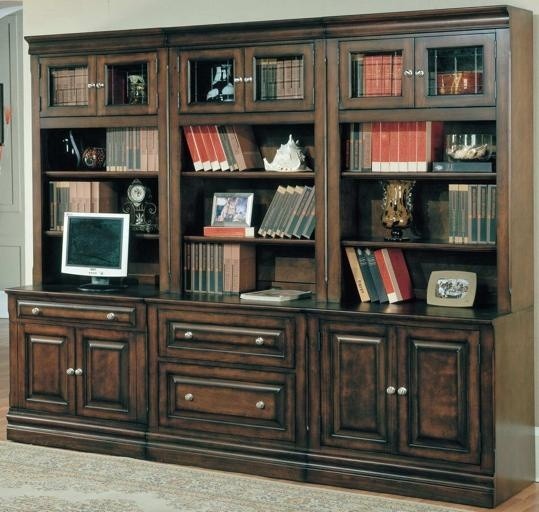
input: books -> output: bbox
[184,54,316,304]
[345,247,414,304]
[342,56,494,173]
[448,185,496,244]
[48,67,159,286]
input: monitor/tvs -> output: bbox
[61,211,131,292]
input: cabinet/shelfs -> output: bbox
[328,5,533,308]
[24,28,168,292]
[305,308,533,506]
[149,298,303,484]
[168,16,328,303]
[5,291,148,461]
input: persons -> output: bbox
[439,281,468,300]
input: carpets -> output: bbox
[0,439,450,511]
